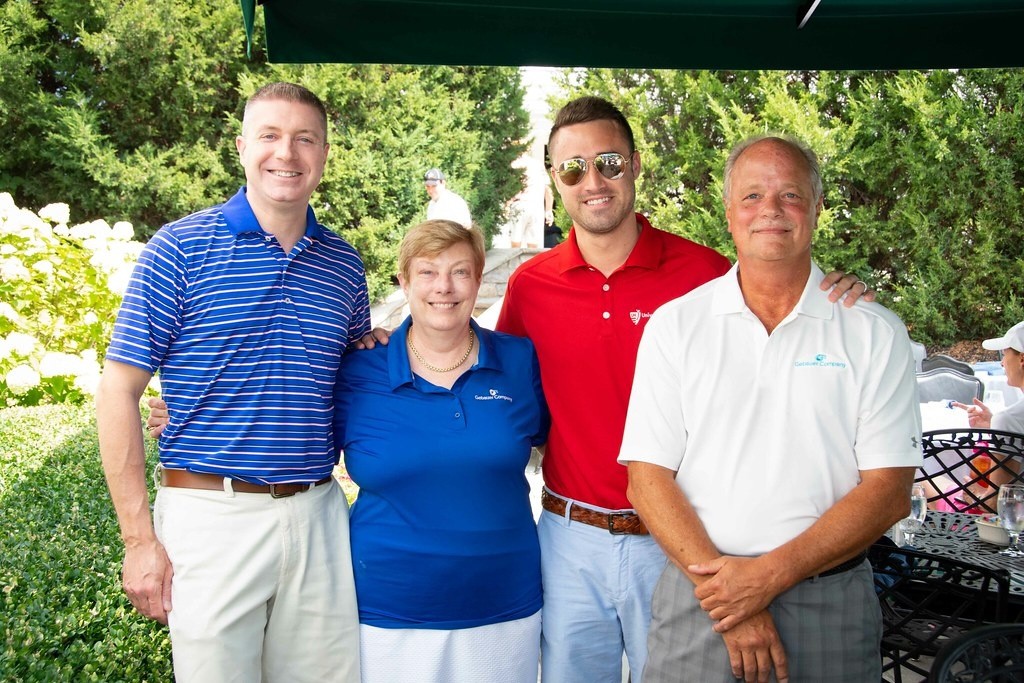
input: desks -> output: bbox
[885,509,1024,598]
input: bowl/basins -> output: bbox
[975,518,1010,546]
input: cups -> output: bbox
[983,391,1005,416]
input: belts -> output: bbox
[157,469,330,499]
[805,546,868,580]
[541,487,652,536]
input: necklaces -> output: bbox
[409,326,473,370]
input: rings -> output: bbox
[857,280,867,292]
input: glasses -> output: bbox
[552,153,636,187]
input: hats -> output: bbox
[982,319,1024,354]
[422,168,446,186]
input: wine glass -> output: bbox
[900,497,927,546]
[997,485,1024,557]
[906,483,925,544]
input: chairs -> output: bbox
[867,544,1010,683]
[909,339,1019,427]
[907,429,1024,516]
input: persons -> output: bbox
[510,152,555,247]
[922,320,1024,537]
[145,221,551,683]
[356,97,876,683]
[617,131,923,683]
[97,82,372,683]
[423,168,473,229]
